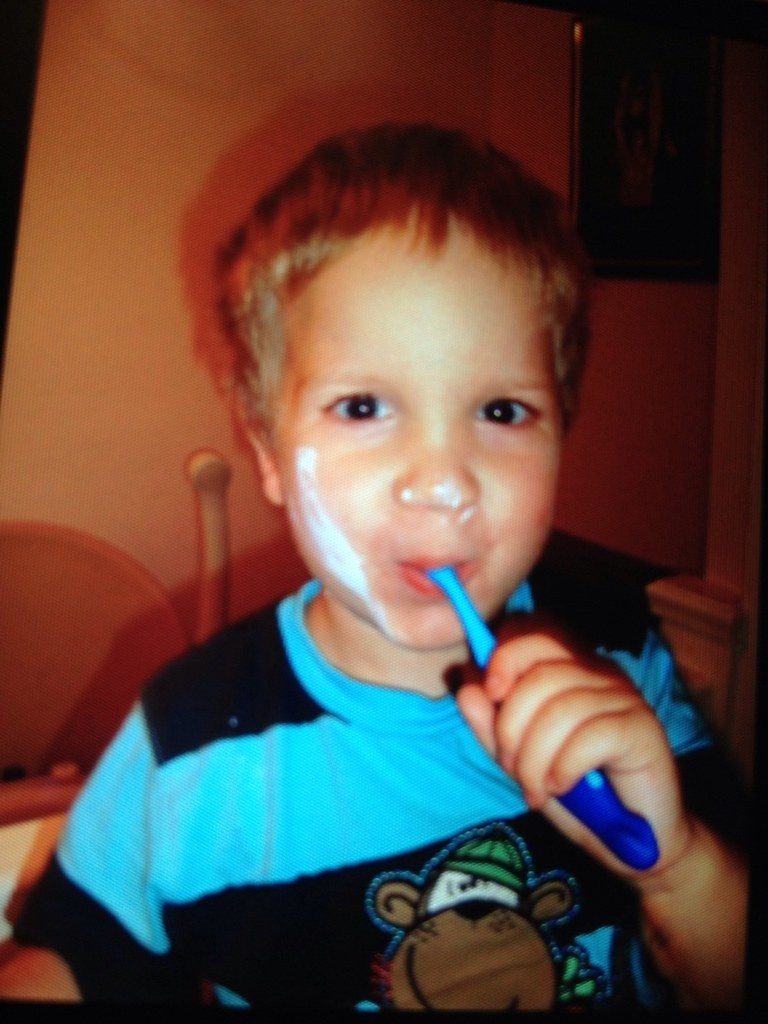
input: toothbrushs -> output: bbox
[427,566,660,870]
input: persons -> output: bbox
[0,118,748,1012]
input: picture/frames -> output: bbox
[571,18,726,285]
